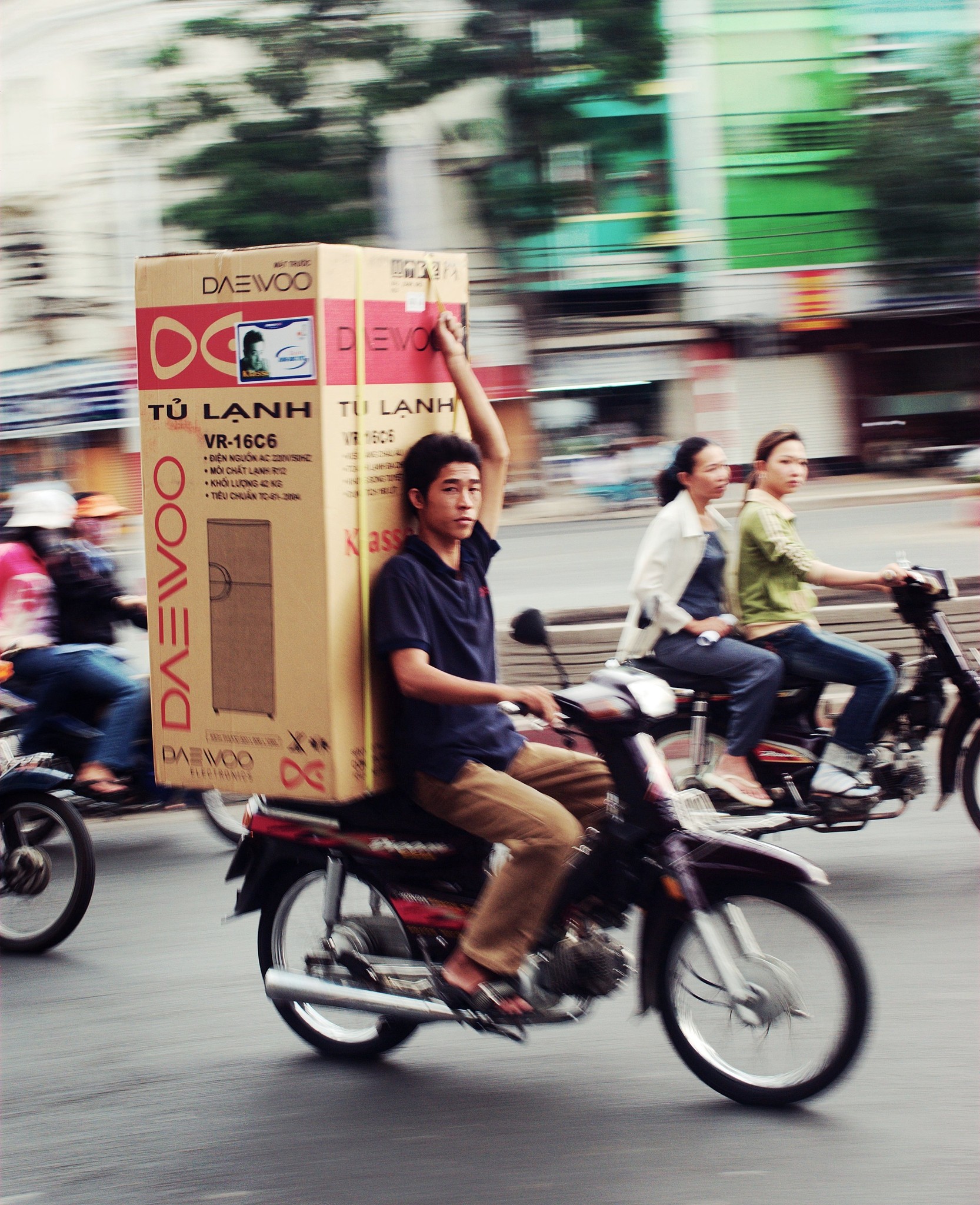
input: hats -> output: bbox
[73,496,132,519]
[3,490,78,529]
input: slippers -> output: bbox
[703,769,776,809]
[435,968,538,1022]
[812,782,878,801]
[72,778,129,796]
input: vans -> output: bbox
[721,295,979,479]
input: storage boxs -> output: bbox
[130,239,472,809]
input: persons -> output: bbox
[733,431,908,798]
[362,309,616,1018]
[2,471,159,794]
[613,437,782,807]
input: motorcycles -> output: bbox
[0,660,98,960]
[650,562,980,844]
[226,608,877,1109]
[0,605,253,845]
